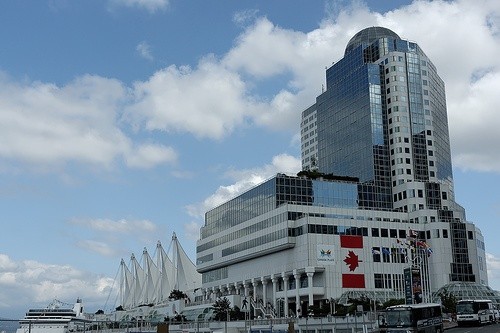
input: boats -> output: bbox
[16,308,93,333]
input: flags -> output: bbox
[372,250,381,255]
[382,249,391,254]
[393,229,433,258]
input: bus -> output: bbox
[377,303,443,333]
[455,299,495,326]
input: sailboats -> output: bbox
[98,231,202,333]
